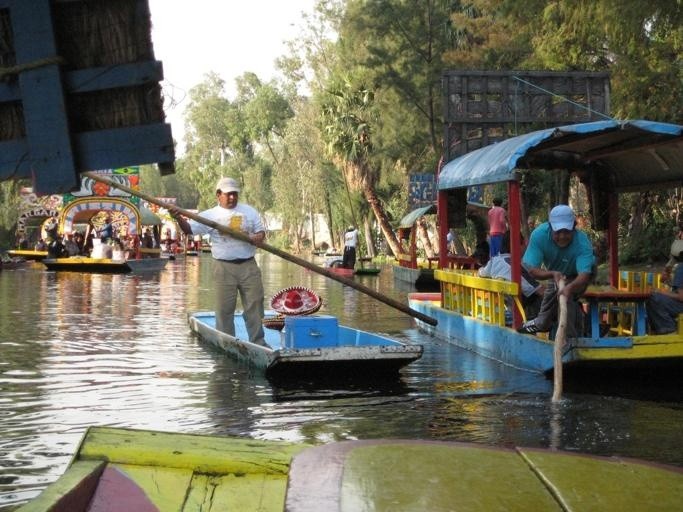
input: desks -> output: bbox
[582,285,651,338]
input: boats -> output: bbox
[188,304,424,377]
[391,201,492,285]
[306,258,381,281]
[6,196,211,271]
[406,118,683,382]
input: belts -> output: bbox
[217,257,253,265]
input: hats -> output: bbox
[548,204,575,232]
[670,240,682,257]
[215,177,240,194]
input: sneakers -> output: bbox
[517,319,552,334]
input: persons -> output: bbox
[646,239,683,334]
[517,204,593,340]
[343,226,358,269]
[167,177,273,351]
[35,239,47,251]
[488,197,506,258]
[96,217,112,242]
